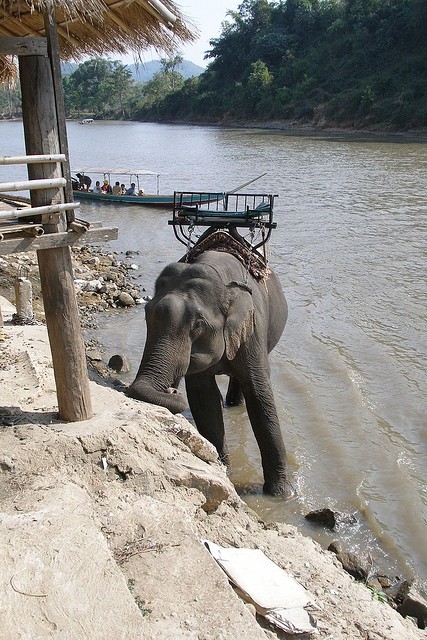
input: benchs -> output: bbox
[167,190,279,235]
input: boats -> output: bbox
[78,119,98,125]
[70,167,267,208]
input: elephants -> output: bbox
[126,247,296,498]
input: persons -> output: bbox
[120,184,126,195]
[101,179,112,195]
[93,180,101,195]
[126,183,136,195]
[113,181,121,196]
[75,172,92,194]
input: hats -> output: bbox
[103,180,108,184]
